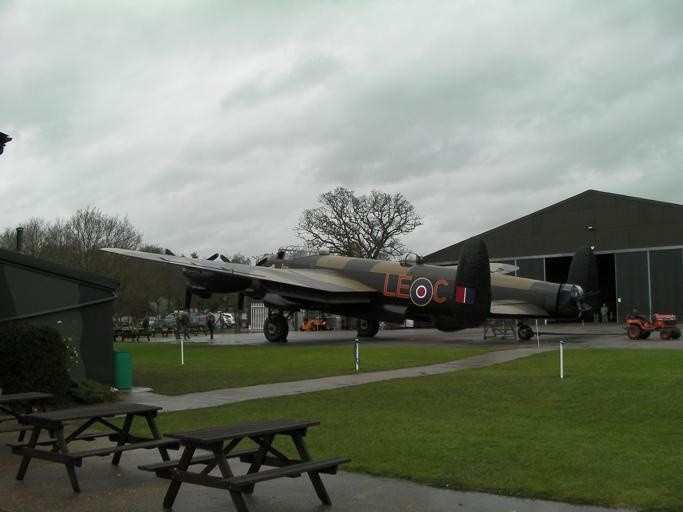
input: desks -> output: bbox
[0,392,181,493]
[159,417,335,510]
[112,329,151,342]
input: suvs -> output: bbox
[625,313,681,340]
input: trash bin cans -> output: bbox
[113,351,133,389]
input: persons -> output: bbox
[160,314,169,337]
[138,311,151,330]
[172,312,181,339]
[112,311,118,343]
[220,316,225,328]
[181,311,191,340]
[599,302,608,324]
[206,312,216,340]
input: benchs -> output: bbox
[138,447,265,476]
[10,431,117,452]
[221,452,351,491]
[112,333,151,342]
[65,436,178,459]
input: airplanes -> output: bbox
[99,237,601,343]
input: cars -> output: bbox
[141,317,159,327]
[166,312,236,329]
